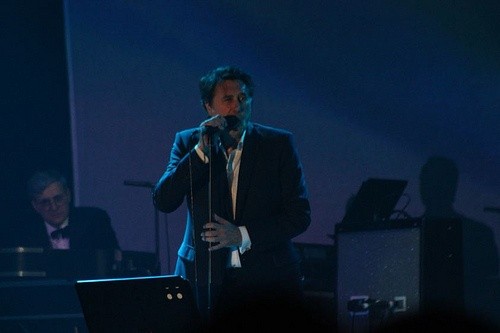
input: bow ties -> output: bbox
[50,226,71,240]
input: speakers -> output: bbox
[338,215,464,333]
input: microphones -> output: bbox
[362,301,401,309]
[196,116,237,133]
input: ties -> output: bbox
[225,148,241,213]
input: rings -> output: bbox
[215,237,219,243]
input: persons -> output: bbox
[153,66,311,333]
[15,168,125,280]
